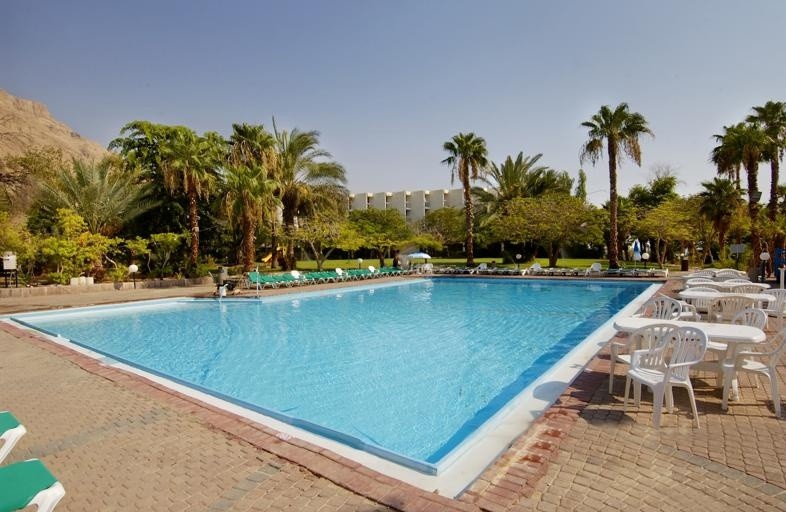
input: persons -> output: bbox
[491,260,496,268]
[379,252,385,268]
[774,237,785,283]
[392,255,400,267]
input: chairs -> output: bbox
[610,322,685,405]
[624,327,707,431]
[627,293,683,355]
[0,457,67,512]
[0,410,29,463]
[699,307,767,390]
[677,267,786,343]
[431,261,670,278]
[235,264,435,290]
[720,328,786,415]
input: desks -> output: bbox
[615,317,765,402]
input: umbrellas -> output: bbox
[632,237,642,262]
[408,251,431,259]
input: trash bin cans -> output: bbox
[680,256,688,271]
[218,266,228,284]
